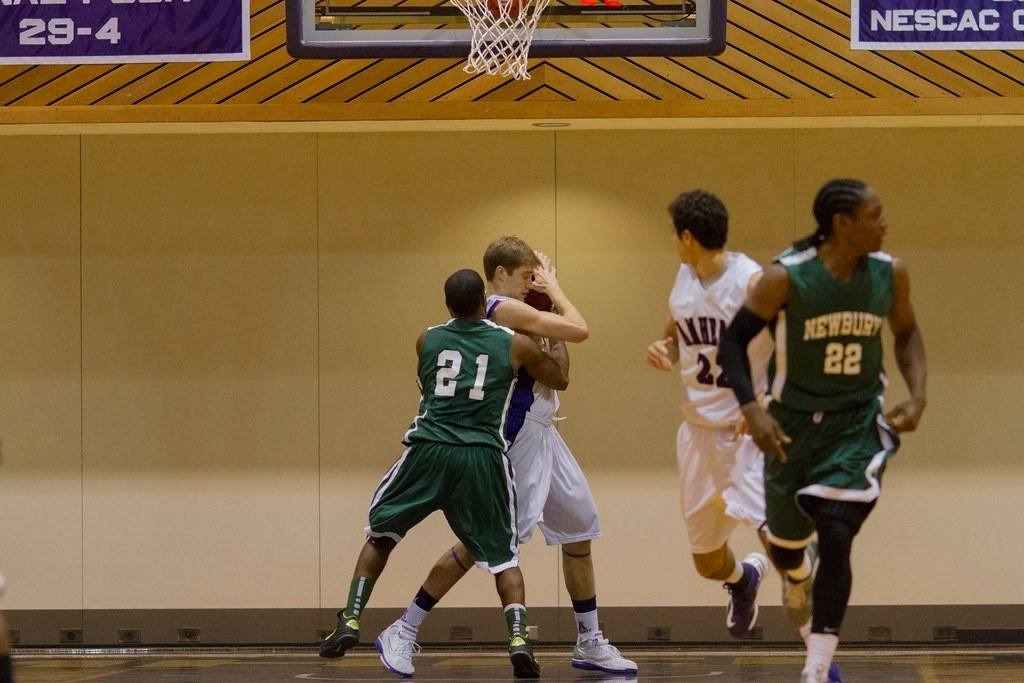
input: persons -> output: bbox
[645,188,843,683]
[315,236,641,674]
[721,177,929,683]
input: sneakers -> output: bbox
[782,539,820,628]
[723,552,769,638]
[571,630,637,674]
[318,608,360,659]
[507,633,541,678]
[375,618,421,678]
